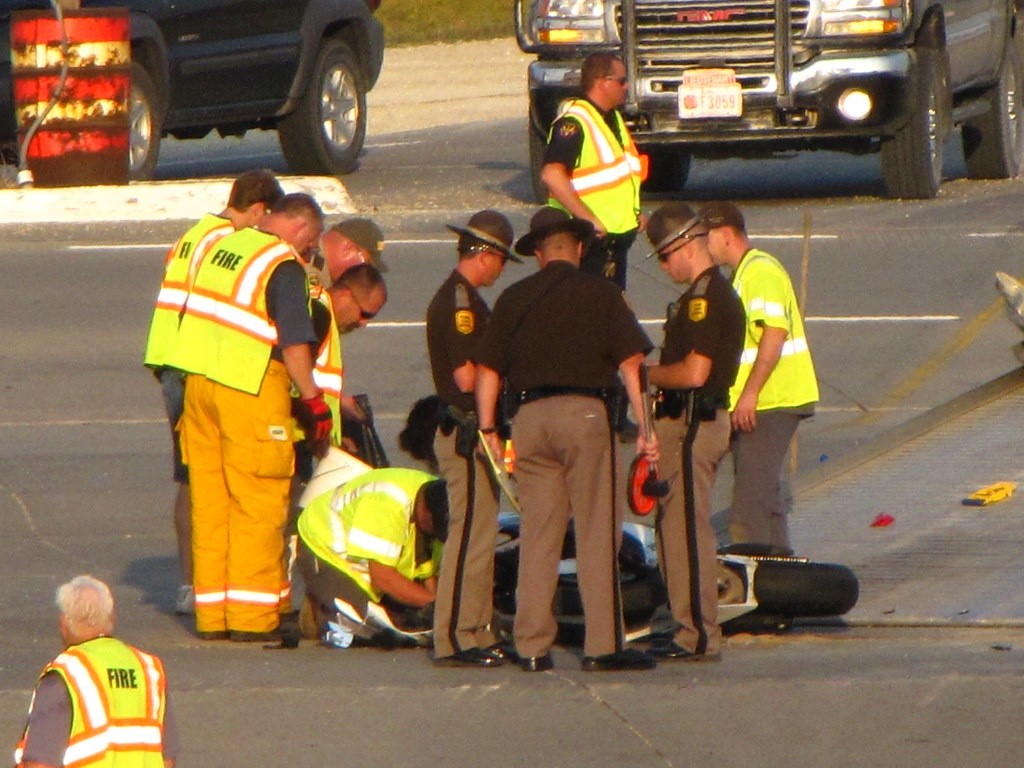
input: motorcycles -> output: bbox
[486,511,862,646]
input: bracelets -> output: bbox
[481,427,495,434]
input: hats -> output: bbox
[335,218,388,275]
[644,201,702,261]
[697,201,745,227]
[428,479,448,542]
[514,207,593,256]
[446,210,524,266]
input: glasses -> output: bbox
[346,286,377,320]
[482,251,510,265]
[656,236,696,262]
[602,75,627,87]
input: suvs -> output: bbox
[514,0,1024,198]
[1,0,386,177]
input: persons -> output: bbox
[639,200,746,662]
[700,199,821,553]
[11,574,182,767]
[474,207,661,671]
[292,465,448,647]
[419,204,520,665]
[139,169,386,648]
[536,52,650,437]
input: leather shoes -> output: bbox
[580,649,657,673]
[484,642,523,664]
[439,648,498,669]
[644,641,723,662]
[517,655,552,669]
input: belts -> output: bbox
[518,387,607,405]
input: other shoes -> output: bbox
[229,624,301,641]
[196,630,229,641]
[175,585,195,615]
